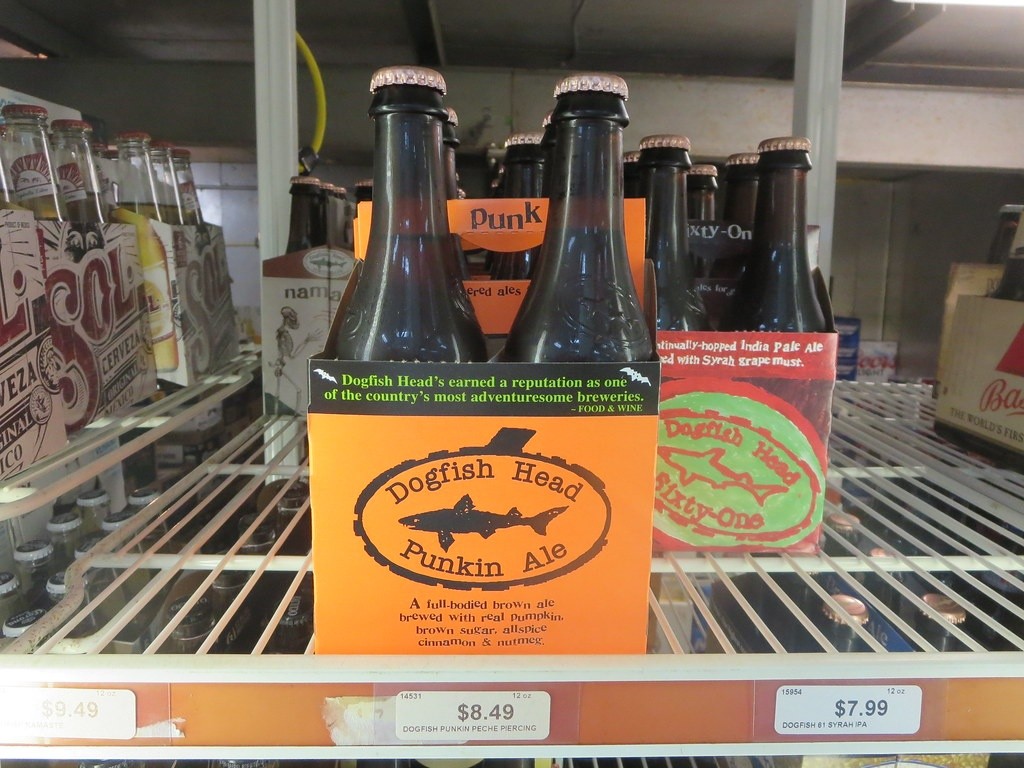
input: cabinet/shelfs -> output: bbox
[0,0,1024,768]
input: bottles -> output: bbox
[2,67,1022,766]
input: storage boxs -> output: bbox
[932,261,1024,470]
[264,195,897,656]
[0,197,236,482]
